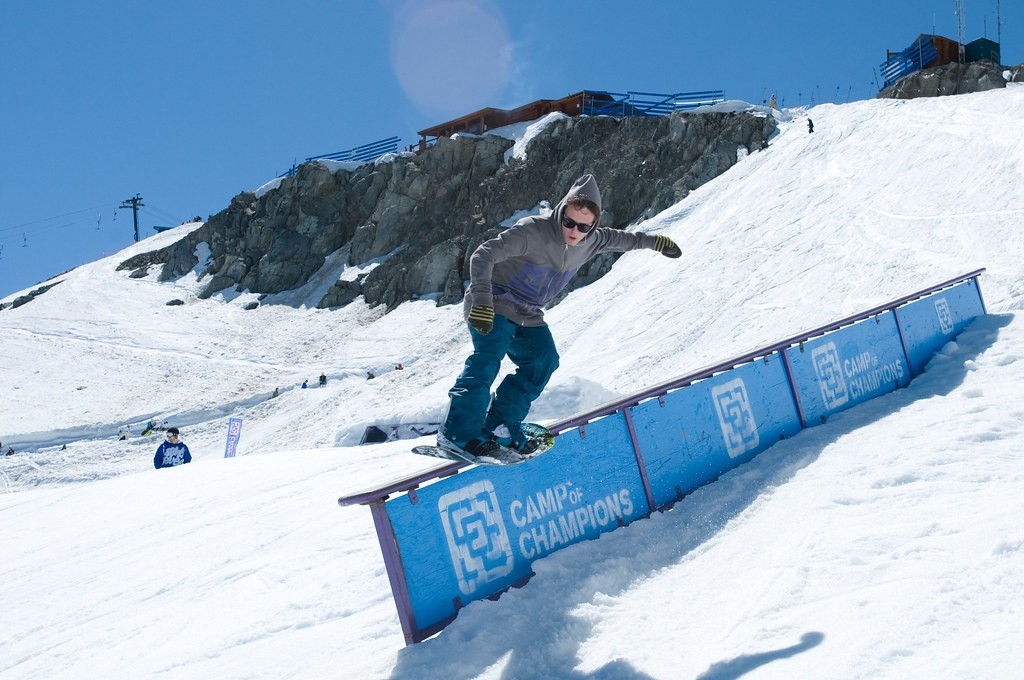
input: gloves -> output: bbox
[654,235,682,258]
[467,305,494,335]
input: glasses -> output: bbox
[166,432,173,438]
[562,213,592,233]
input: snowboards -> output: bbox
[411,419,556,467]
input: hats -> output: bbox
[168,428,179,434]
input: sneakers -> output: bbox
[490,422,541,455]
[436,425,501,459]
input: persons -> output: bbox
[154,428,192,469]
[5,446,14,457]
[769,94,776,108]
[319,372,326,386]
[302,380,308,389]
[807,118,814,134]
[398,364,403,370]
[437,173,682,457]
[366,372,375,380]
[273,388,279,397]
[148,419,154,429]
